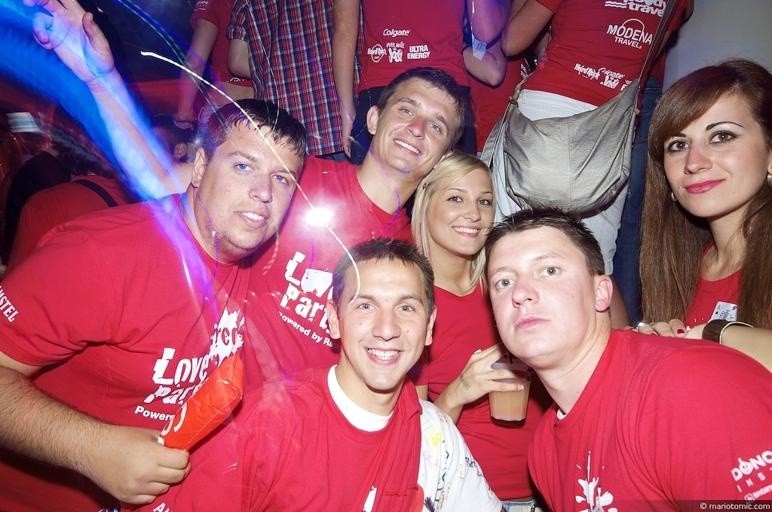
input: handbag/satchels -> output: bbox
[505,78,642,214]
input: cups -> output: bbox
[488,359,534,422]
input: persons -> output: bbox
[1,1,771,512]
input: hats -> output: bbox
[7,111,41,134]
